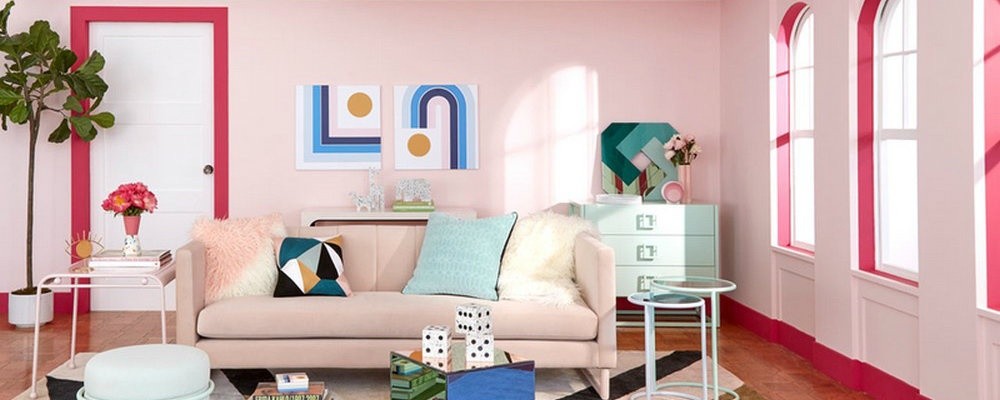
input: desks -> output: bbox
[300,207,478,227]
[389,343,535,400]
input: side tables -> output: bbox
[628,276,739,400]
[29,249,177,400]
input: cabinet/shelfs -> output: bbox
[567,200,721,327]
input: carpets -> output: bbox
[12,350,769,400]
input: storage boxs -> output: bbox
[275,373,310,393]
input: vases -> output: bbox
[123,216,141,235]
[678,165,692,203]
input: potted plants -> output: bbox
[1,0,116,329]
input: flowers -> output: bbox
[662,133,702,165]
[100,182,158,217]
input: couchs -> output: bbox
[174,223,617,400]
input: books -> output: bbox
[252,372,330,400]
[88,250,172,268]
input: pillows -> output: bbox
[401,211,518,301]
[268,233,352,298]
[188,212,288,306]
[497,211,584,306]
